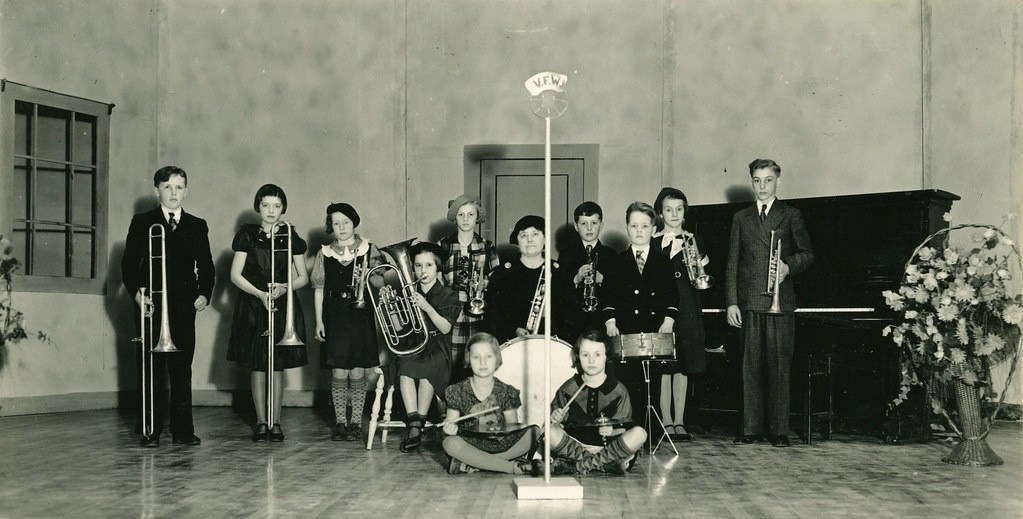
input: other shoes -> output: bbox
[347,423,362,441]
[141,438,159,447]
[771,434,800,446]
[173,434,201,445]
[664,425,677,442]
[734,435,765,443]
[399,425,427,453]
[676,424,693,442]
[550,459,580,475]
[254,423,268,442]
[449,458,482,474]
[603,458,626,475]
[269,424,284,442]
[514,457,545,477]
[332,423,347,441]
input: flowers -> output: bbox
[883,210,1023,408]
[0,233,56,348]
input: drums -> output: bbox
[608,332,678,363]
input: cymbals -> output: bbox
[459,423,532,437]
[573,411,634,427]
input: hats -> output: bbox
[447,194,476,221]
[409,242,449,263]
[509,215,545,245]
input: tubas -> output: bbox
[365,236,429,357]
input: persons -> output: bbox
[442,333,543,475]
[651,187,698,443]
[725,159,815,447]
[602,201,676,337]
[487,216,562,345]
[556,202,618,344]
[227,184,308,443]
[378,242,461,453]
[310,202,387,442]
[437,194,498,386]
[534,331,644,476]
[121,165,215,447]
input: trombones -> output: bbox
[131,222,184,440]
[261,221,307,431]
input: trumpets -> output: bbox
[678,228,715,290]
[464,238,488,315]
[761,229,785,316]
[578,244,601,313]
[347,248,367,309]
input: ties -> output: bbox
[169,213,177,231]
[760,204,767,224]
[636,251,645,274]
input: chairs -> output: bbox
[366,366,447,450]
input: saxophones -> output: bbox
[524,262,552,335]
